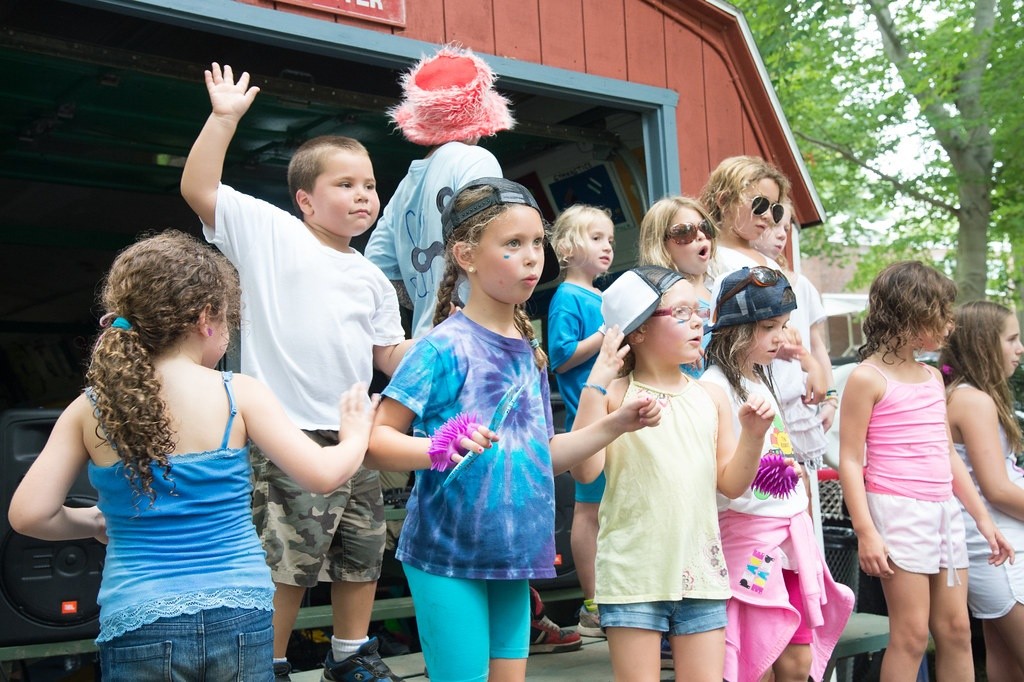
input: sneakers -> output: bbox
[529,586,583,653]
[320,637,403,682]
[273,662,291,682]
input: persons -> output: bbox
[570,263,774,682]
[697,267,856,682]
[839,259,1015,682]
[366,178,661,681]
[639,195,804,380]
[364,39,583,657]
[178,62,419,682]
[700,155,827,404]
[938,301,1024,682]
[545,204,615,638]
[7,223,381,682]
[750,194,844,470]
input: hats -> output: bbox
[441,177,543,252]
[387,42,517,144]
[600,265,685,337]
[703,266,797,335]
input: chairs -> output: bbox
[822,526,929,682]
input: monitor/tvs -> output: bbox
[541,159,636,232]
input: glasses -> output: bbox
[663,219,714,246]
[651,305,710,322]
[712,266,790,322]
[741,190,784,224]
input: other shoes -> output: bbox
[661,635,671,653]
[576,604,606,637]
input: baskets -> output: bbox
[823,526,860,613]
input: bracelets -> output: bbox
[820,389,839,409]
[581,382,606,395]
